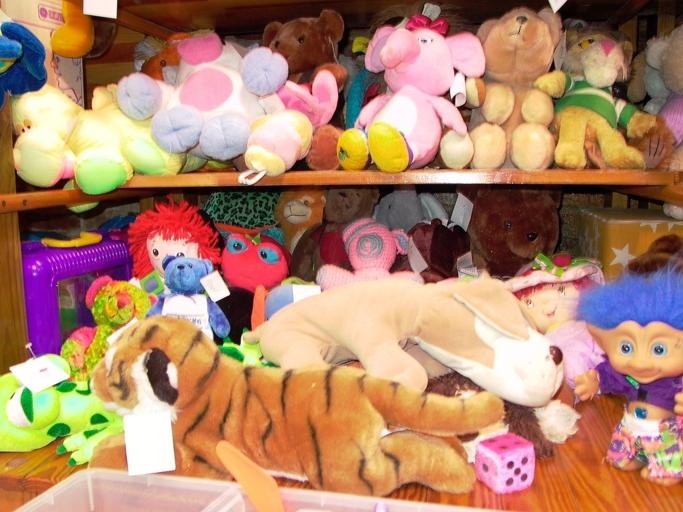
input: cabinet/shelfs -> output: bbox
[1,0,682,512]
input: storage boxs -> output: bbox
[213,483,503,512]
[14,469,238,511]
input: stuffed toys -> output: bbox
[0,0,683,512]
